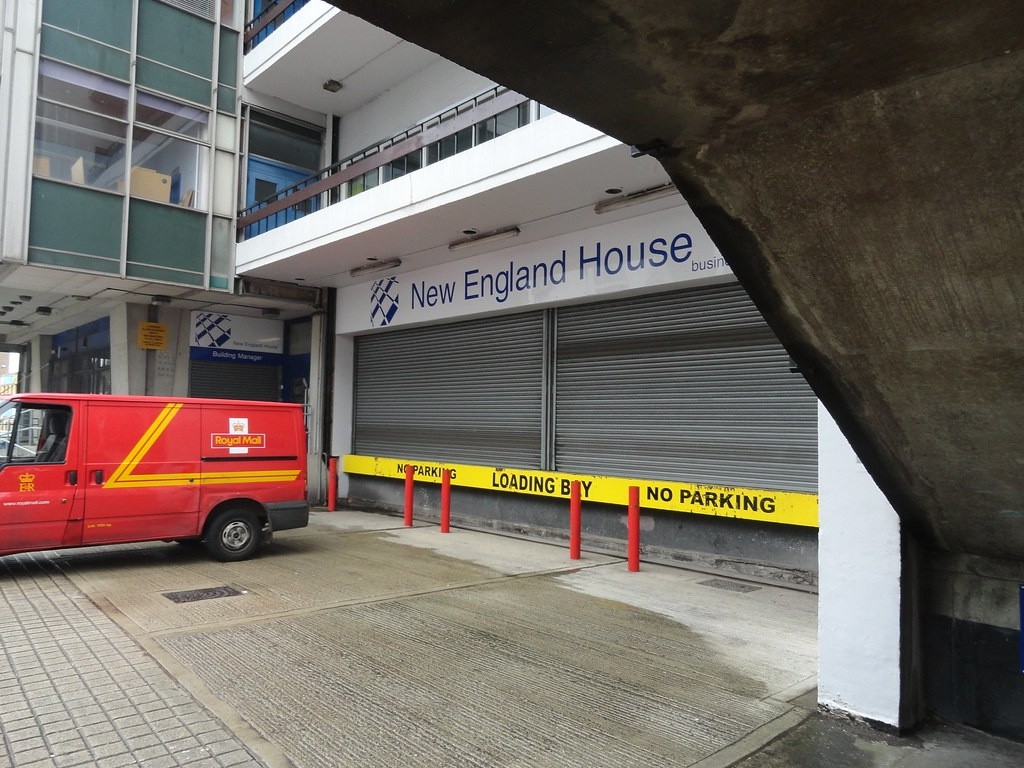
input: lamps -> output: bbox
[37,307,51,315]
[152,295,171,306]
[350,258,401,277]
[8,320,23,328]
[595,183,679,214]
[323,80,343,93]
[262,309,279,319]
[72,295,91,301]
[449,226,521,252]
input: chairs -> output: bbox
[34,417,63,462]
[48,420,71,461]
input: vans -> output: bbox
[0,393,310,562]
